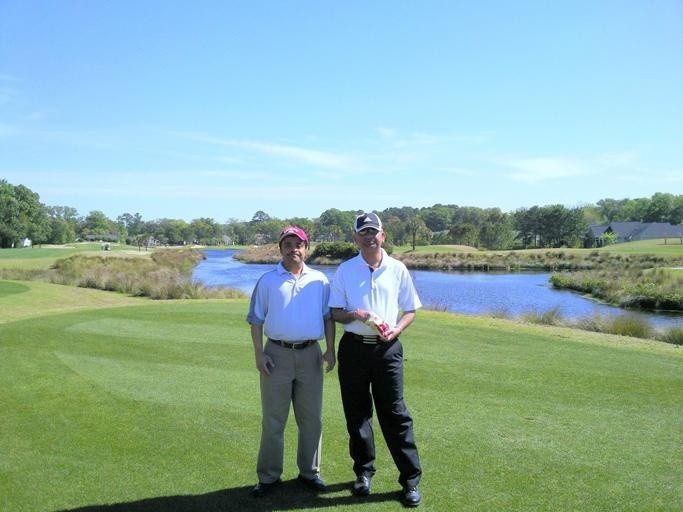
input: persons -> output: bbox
[244,226,336,496]
[326,211,424,508]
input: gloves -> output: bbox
[364,313,388,338]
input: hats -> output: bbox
[352,212,381,231]
[278,226,307,248]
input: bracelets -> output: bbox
[394,325,402,331]
[352,309,358,319]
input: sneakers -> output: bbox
[296,474,325,491]
[251,480,279,496]
[353,476,371,495]
[402,487,420,506]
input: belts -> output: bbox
[353,335,382,344]
[271,340,315,349]
